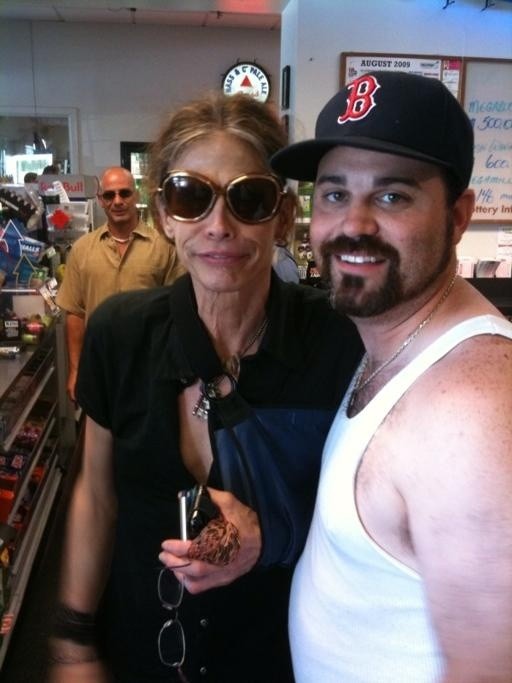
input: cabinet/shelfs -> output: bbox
[1,299,63,678]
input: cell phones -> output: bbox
[177,489,192,543]
[189,484,221,542]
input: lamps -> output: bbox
[27,21,49,156]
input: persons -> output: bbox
[269,69,512,682]
[40,90,364,682]
[54,165,189,412]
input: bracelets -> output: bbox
[51,600,102,646]
[44,655,107,669]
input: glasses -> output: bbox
[156,560,195,682]
[97,189,134,201]
[154,171,289,223]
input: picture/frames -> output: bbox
[281,64,290,110]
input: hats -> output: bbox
[268,69,474,195]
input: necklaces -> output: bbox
[190,310,272,418]
[350,267,466,415]
